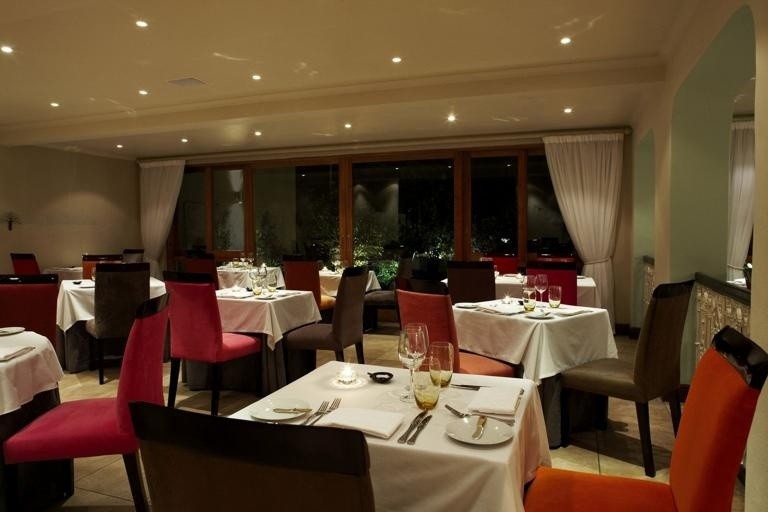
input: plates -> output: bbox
[441,415,514,448]
[525,312,552,319]
[455,303,476,308]
[249,398,311,421]
[0,326,26,336]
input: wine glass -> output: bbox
[534,274,547,307]
[233,249,279,296]
[395,319,430,397]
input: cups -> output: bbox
[409,355,443,413]
[90,267,96,281]
[424,340,453,389]
[522,290,536,311]
[520,275,534,295]
[549,285,562,309]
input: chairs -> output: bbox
[82,259,154,386]
[9,255,123,274]
[396,287,513,376]
[162,269,264,418]
[525,260,578,307]
[127,399,379,510]
[477,255,578,277]
[178,252,220,290]
[279,252,337,311]
[360,255,413,332]
[446,260,498,305]
[524,323,767,511]
[284,264,371,382]
[0,291,173,511]
[558,276,697,478]
[2,273,60,353]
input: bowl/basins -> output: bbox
[372,371,393,383]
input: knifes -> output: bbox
[470,416,488,439]
[406,415,433,446]
[272,408,312,414]
[397,408,428,443]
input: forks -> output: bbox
[306,396,342,427]
[444,405,515,425]
[300,400,329,426]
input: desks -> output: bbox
[182,284,322,394]
[450,296,619,451]
[55,277,171,374]
[226,360,554,511]
[442,273,605,308]
[1,325,75,510]
